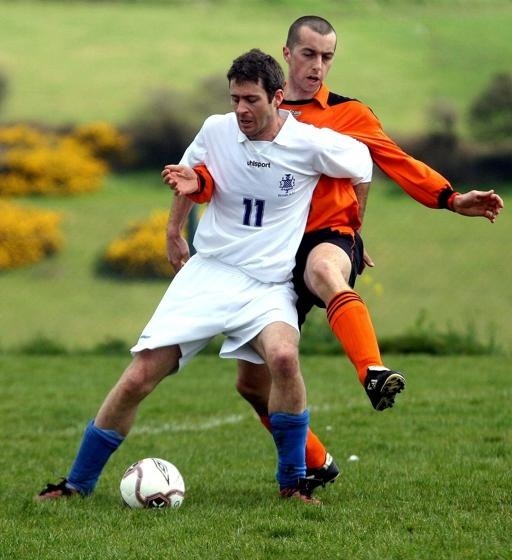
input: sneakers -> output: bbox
[34,482,78,500]
[364,366,405,411]
[279,453,340,508]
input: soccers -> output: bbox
[119,456,185,510]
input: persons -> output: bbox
[163,13,505,506]
[33,46,377,511]
